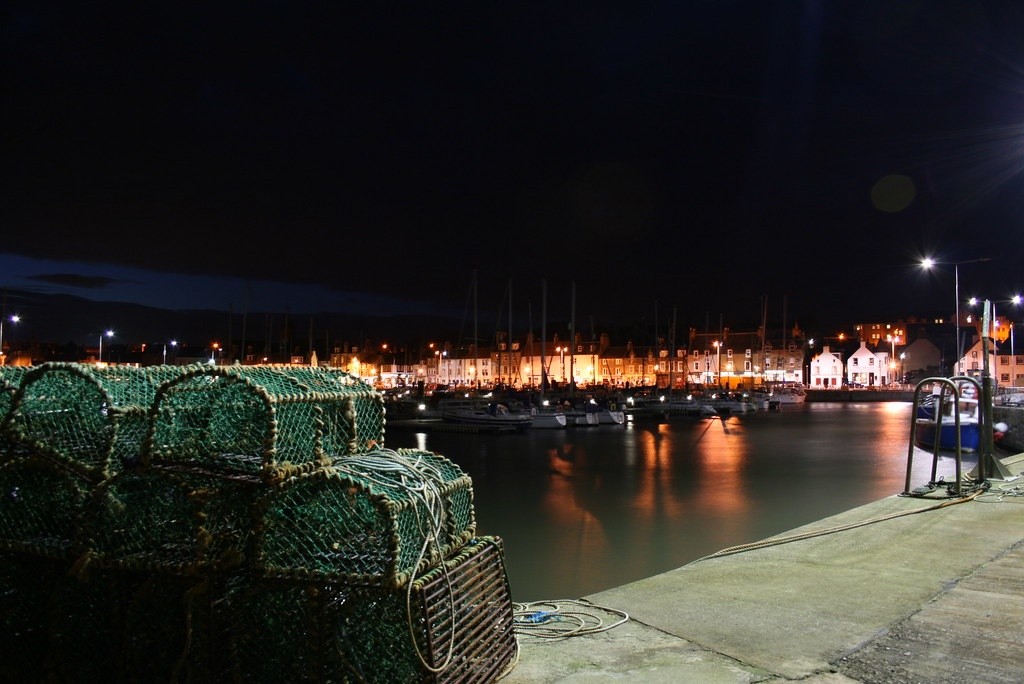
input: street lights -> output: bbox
[967,295,1023,403]
[919,254,998,377]
[712,341,723,389]
[0,315,20,354]
[98,331,115,362]
[210,343,219,360]
[163,342,177,366]
[887,336,899,383]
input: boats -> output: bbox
[910,365,1007,454]
[373,273,810,430]
